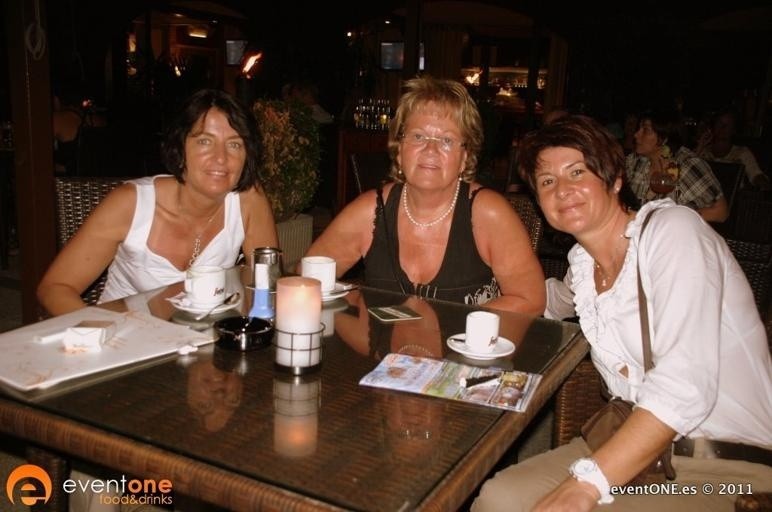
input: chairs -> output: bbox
[550,316,772,510]
[55,172,133,310]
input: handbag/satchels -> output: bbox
[582,399,676,493]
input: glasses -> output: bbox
[383,420,433,440]
[401,128,462,151]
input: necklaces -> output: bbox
[585,232,630,291]
[174,193,232,270]
[400,177,462,228]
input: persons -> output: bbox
[538,109,577,124]
[33,89,285,320]
[621,113,732,224]
[698,108,772,194]
[623,110,640,154]
[294,76,548,321]
[465,113,770,509]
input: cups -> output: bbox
[465,310,500,354]
[190,265,226,306]
[300,256,336,298]
[251,246,284,288]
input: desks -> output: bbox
[1,267,592,511]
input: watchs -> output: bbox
[568,456,616,505]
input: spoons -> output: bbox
[194,291,241,321]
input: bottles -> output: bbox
[356,119,392,130]
[356,96,393,118]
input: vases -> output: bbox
[276,211,314,274]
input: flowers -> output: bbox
[252,100,324,225]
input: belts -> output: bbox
[673,437,772,467]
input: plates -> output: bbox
[317,278,353,301]
[446,331,516,362]
[170,290,241,312]
